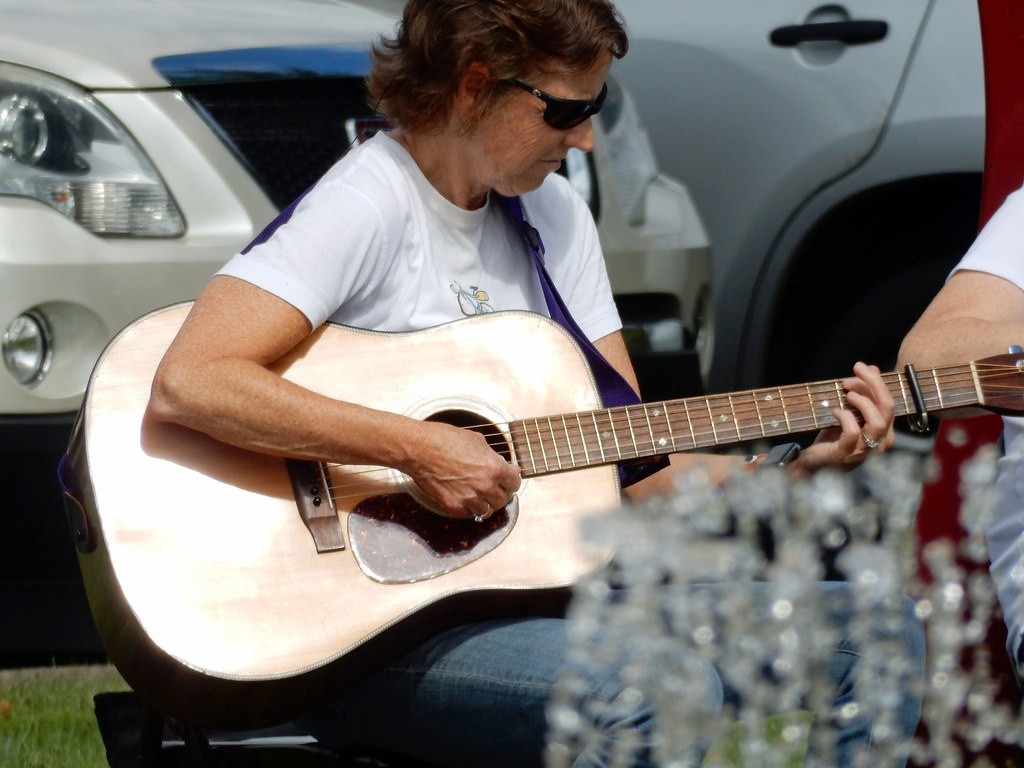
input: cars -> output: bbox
[0,1,988,574]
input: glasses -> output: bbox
[496,78,608,130]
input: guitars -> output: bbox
[58,297,1024,730]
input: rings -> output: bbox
[862,432,880,449]
[472,504,491,523]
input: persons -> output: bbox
[146,0,895,766]
[893,186,1022,692]
